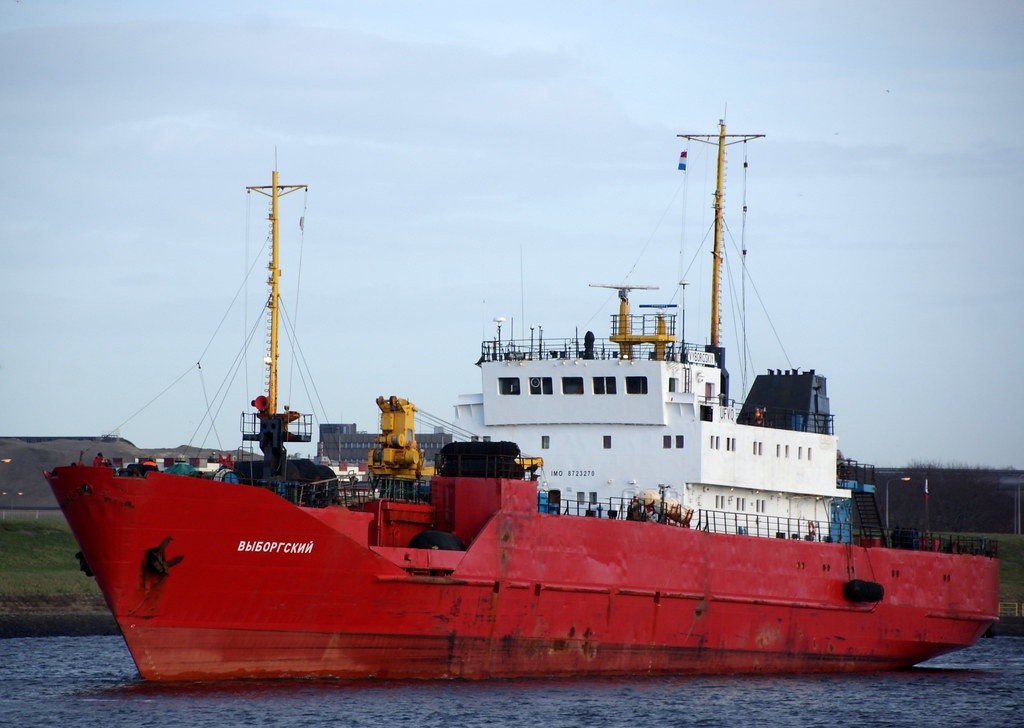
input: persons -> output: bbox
[92,452,108,467]
[890,526,920,549]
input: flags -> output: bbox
[924,478,930,503]
